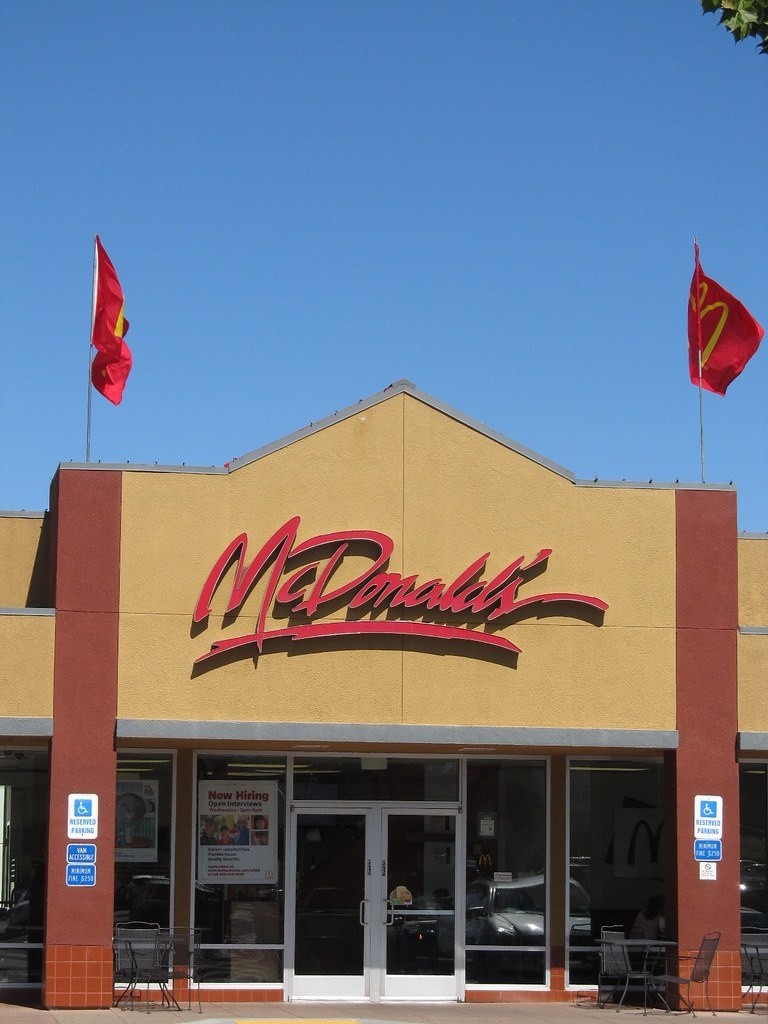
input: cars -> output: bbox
[463,871,595,983]
[126,873,230,921]
[294,885,455,972]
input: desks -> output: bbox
[592,938,679,1017]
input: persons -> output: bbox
[200,816,249,845]
[254,833,264,845]
[630,894,665,940]
[253,816,266,829]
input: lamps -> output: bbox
[390,880,416,906]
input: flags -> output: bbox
[688,243,764,397]
[91,237,133,406]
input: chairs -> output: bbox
[113,921,205,1014]
[592,924,768,1019]
